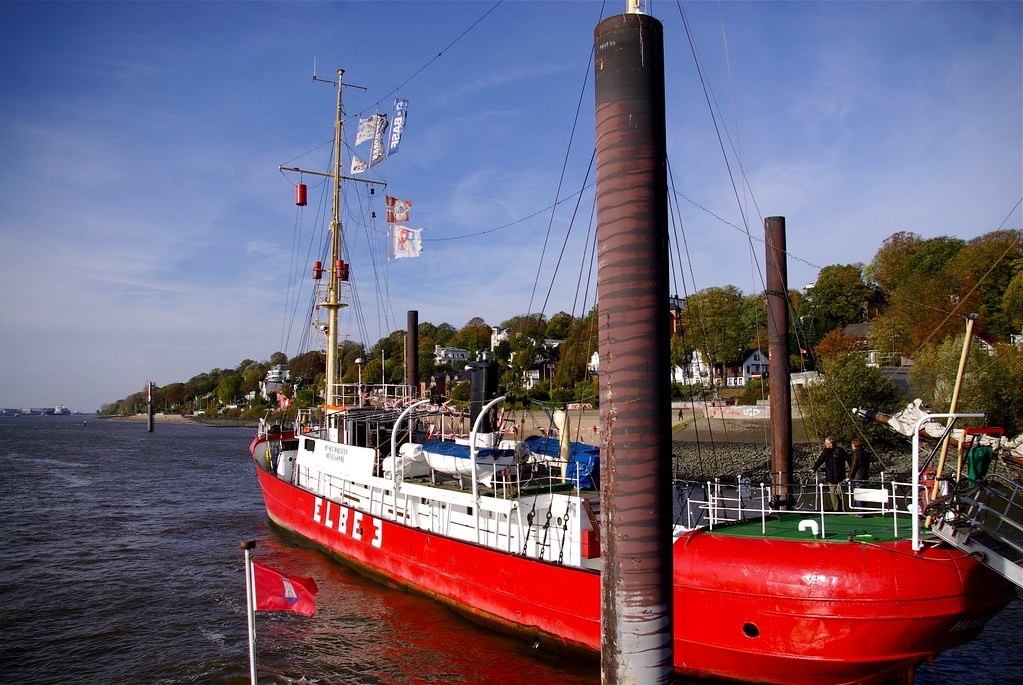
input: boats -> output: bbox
[249,56,1023,685]
[0,404,72,416]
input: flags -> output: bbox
[388,97,410,157]
[356,116,375,146]
[901,357,914,366]
[385,222,423,261]
[371,113,390,168]
[350,154,367,175]
[386,194,411,223]
[250,560,319,618]
[801,349,806,353]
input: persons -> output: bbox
[809,436,852,517]
[360,385,373,406]
[844,438,870,518]
[678,409,684,420]
